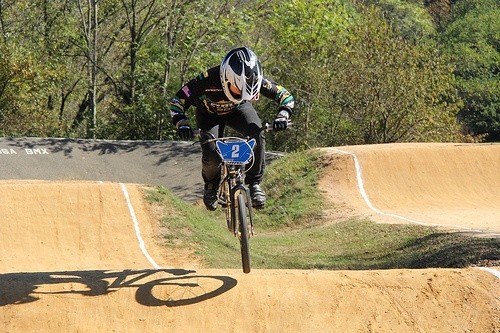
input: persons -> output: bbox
[170,47,294,211]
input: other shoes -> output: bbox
[252,195,266,206]
[203,189,217,207]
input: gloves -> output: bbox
[272,116,287,132]
[177,124,195,141]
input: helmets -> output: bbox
[219,46,263,104]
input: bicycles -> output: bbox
[194,122,291,273]
